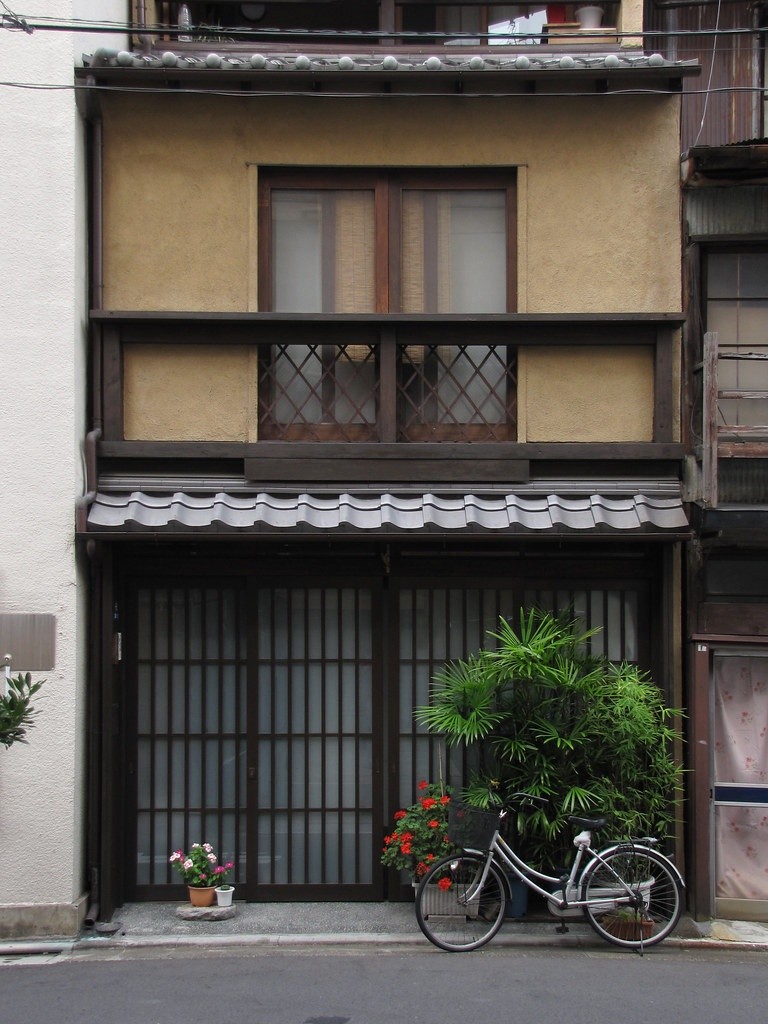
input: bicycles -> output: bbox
[413,791,686,960]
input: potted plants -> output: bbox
[575,0,605,29]
[599,808,655,940]
[215,885,235,907]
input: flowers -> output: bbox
[170,843,235,888]
[381,779,467,895]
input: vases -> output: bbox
[187,884,217,907]
[411,875,484,920]
[544,864,587,895]
[501,871,529,919]
